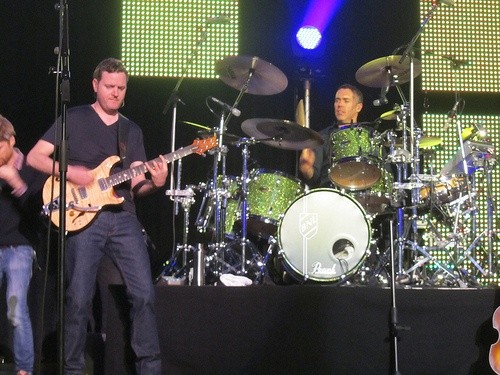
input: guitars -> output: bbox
[42,135,219,232]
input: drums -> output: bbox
[197,174,243,239]
[329,125,385,191]
[236,168,309,238]
[333,168,394,206]
[418,173,473,203]
[277,188,372,286]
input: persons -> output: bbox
[300,83,417,285]
[0,57,168,375]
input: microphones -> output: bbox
[209,95,241,117]
[372,98,389,107]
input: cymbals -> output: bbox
[355,55,422,87]
[241,118,326,150]
[215,54,288,96]
[386,135,443,150]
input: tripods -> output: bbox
[368,0,500,289]
[153,26,272,286]
